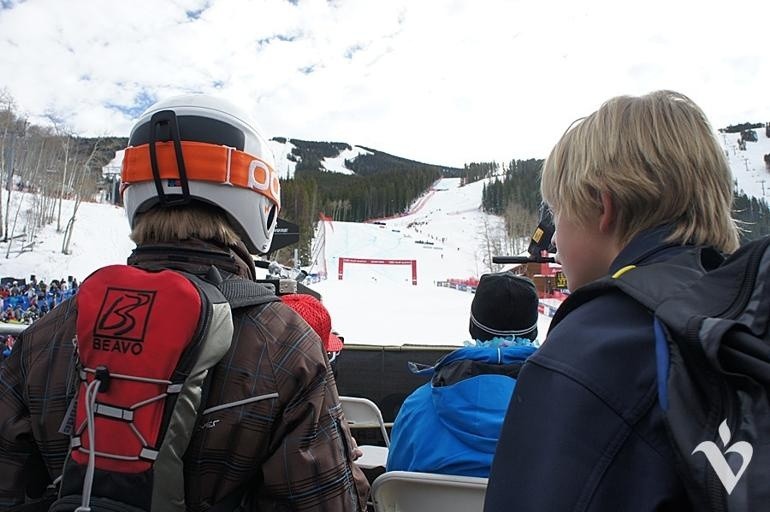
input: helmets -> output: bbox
[117,94,284,256]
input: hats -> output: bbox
[468,271,540,344]
[279,291,347,354]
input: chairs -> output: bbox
[371,471,489,512]
[338,396,390,506]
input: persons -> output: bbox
[0,88,373,511]
[280,292,364,459]
[381,271,542,478]
[482,89,770,512]
[0,273,82,360]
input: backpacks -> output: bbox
[547,234,770,511]
